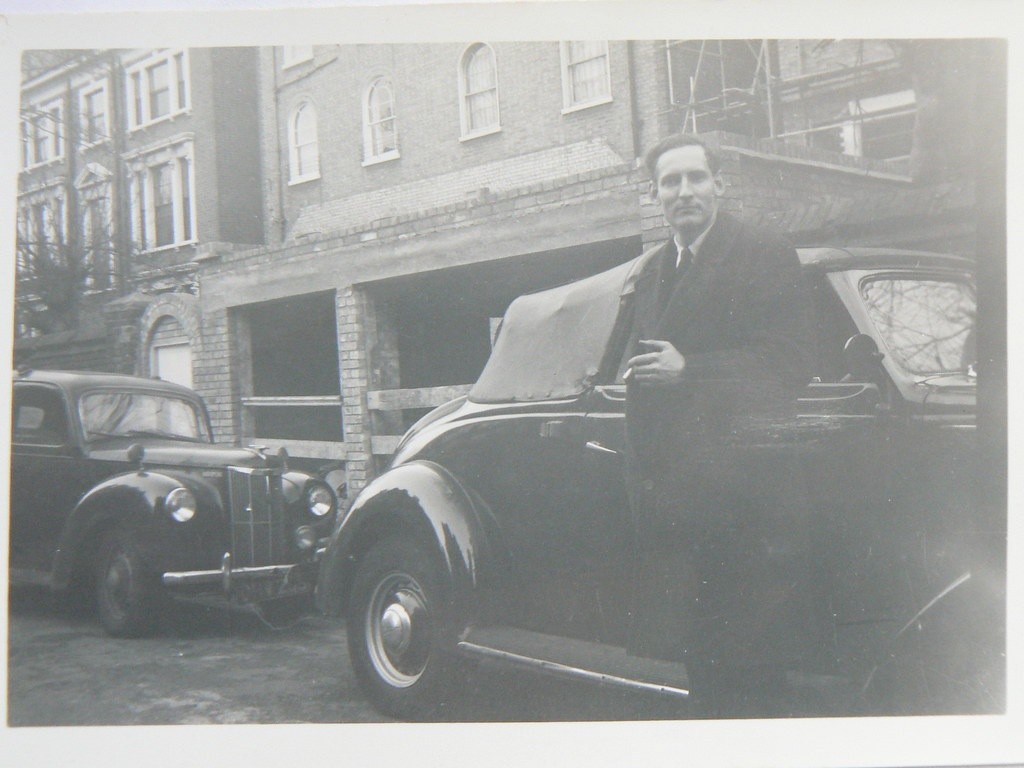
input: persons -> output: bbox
[621,132,829,720]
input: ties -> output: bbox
[666,249,692,302]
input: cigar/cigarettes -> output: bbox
[623,367,632,380]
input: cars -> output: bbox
[12,365,337,638]
[315,244,978,718]
